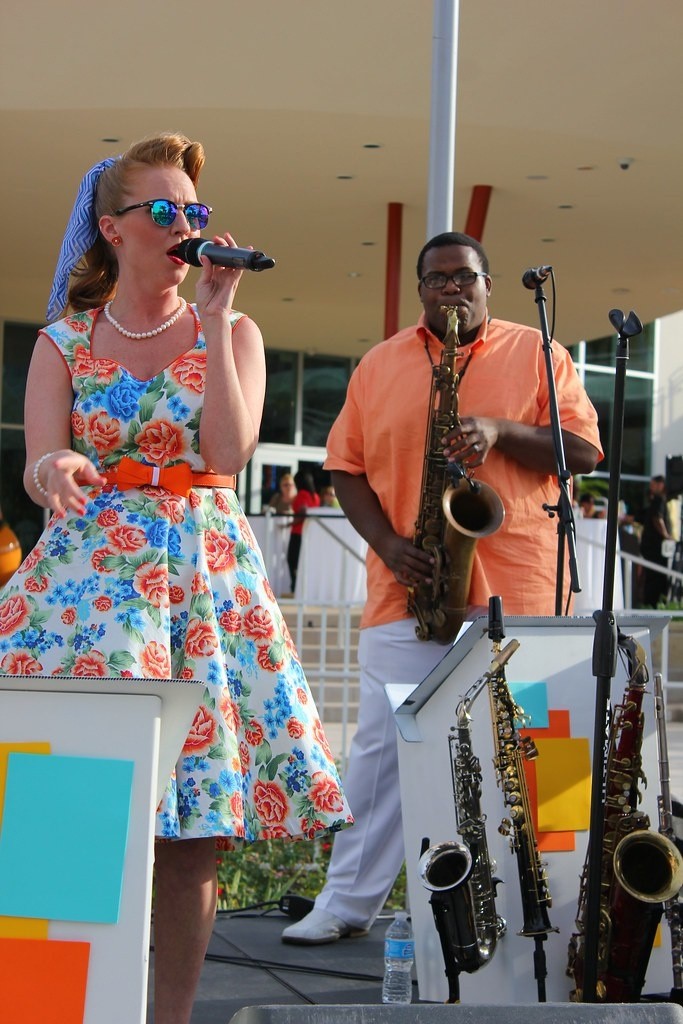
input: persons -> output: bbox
[281,230,605,943]
[269,472,341,599]
[572,474,675,609]
[0,133,355,1024]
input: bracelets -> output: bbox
[34,452,55,497]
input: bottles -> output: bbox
[381,911,415,1005]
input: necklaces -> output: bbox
[104,296,187,339]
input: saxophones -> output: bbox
[406,302,683,1004]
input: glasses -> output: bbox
[418,272,487,289]
[108,197,213,229]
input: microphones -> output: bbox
[179,238,275,272]
[522,266,553,290]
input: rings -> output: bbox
[473,443,480,453]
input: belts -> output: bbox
[72,456,234,499]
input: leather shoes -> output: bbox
[282,908,368,943]
